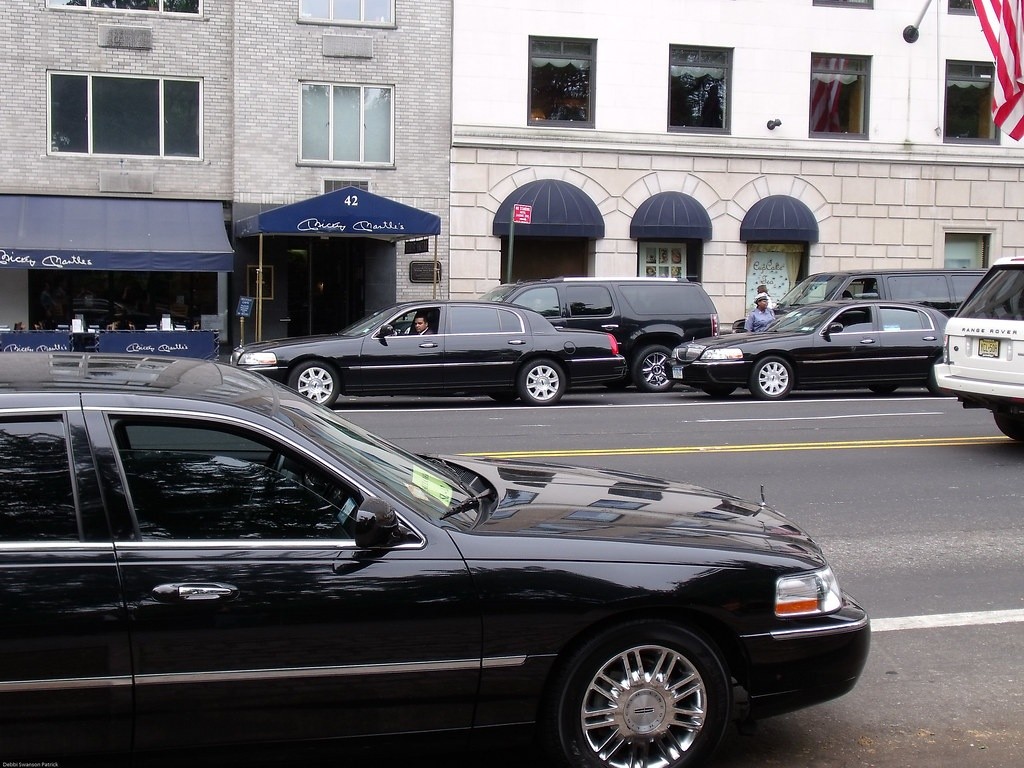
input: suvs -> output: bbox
[927,255,1024,441]
[732,267,991,333]
[474,273,720,394]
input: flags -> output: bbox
[809,51,849,134]
[971,0,1024,142]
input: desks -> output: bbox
[98,329,221,360]
[1,329,74,353]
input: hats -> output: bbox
[754,292,769,304]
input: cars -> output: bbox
[229,299,628,409]
[0,350,871,768]
[663,299,960,401]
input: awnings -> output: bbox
[235,186,441,238]
[492,178,606,238]
[740,194,820,243]
[630,191,713,239]
[0,193,236,272]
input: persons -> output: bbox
[389,313,435,336]
[744,284,776,333]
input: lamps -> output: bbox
[766,118,782,130]
